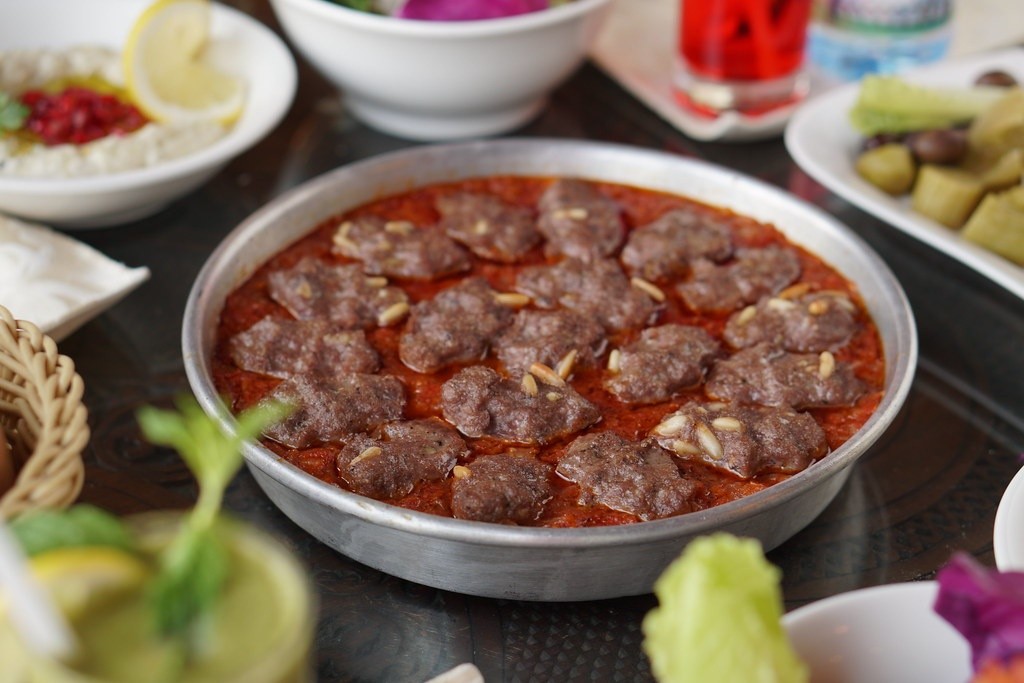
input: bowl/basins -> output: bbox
[269,1,611,142]
[0,0,298,229]
[182,137,916,601]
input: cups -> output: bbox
[667,1,813,120]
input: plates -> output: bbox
[583,0,1024,144]
[785,46,1024,307]
[992,467,1024,574]
[1,214,149,346]
[775,582,1024,683]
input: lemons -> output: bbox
[125,0,247,122]
[25,551,153,620]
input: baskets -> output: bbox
[0,303,92,526]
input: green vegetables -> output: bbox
[11,398,298,633]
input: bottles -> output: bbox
[0,509,315,683]
[826,0,956,53]
